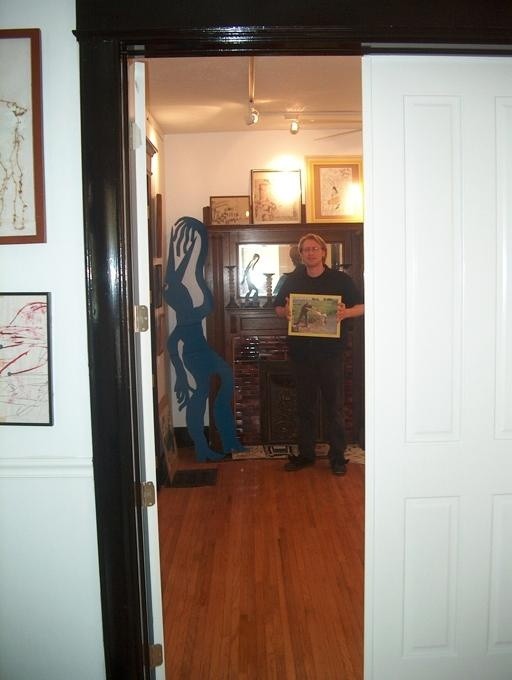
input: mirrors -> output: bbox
[238,243,343,298]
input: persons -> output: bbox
[273,246,304,296]
[273,233,365,474]
[294,301,314,329]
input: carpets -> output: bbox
[232,446,364,467]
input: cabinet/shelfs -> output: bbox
[203,207,364,446]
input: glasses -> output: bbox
[301,247,320,252]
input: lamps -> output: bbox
[247,110,261,129]
[290,120,300,135]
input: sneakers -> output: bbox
[331,454,345,474]
[284,455,317,471]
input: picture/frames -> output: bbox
[289,294,341,339]
[158,394,178,487]
[1,29,46,246]
[2,294,54,426]
[209,155,363,224]
